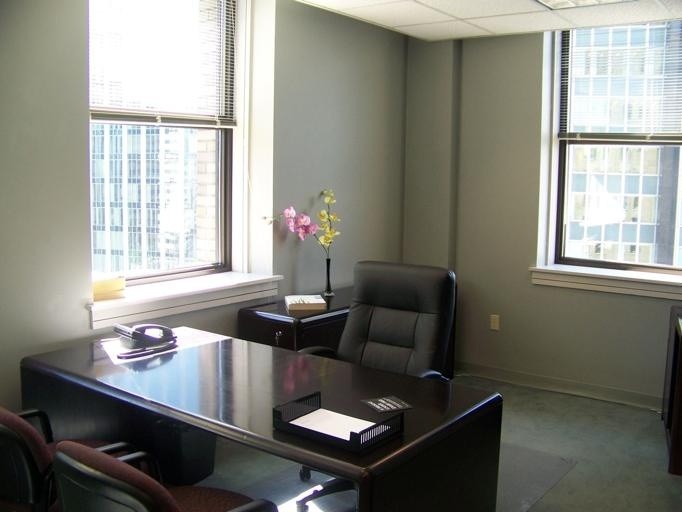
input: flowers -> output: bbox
[261,186,341,254]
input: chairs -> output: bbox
[0,400,276,509]
[288,253,458,512]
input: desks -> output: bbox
[17,322,504,511]
[237,284,355,351]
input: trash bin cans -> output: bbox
[137,407,216,487]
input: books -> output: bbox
[285,294,328,312]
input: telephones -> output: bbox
[113,324,174,349]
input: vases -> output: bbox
[323,258,333,294]
